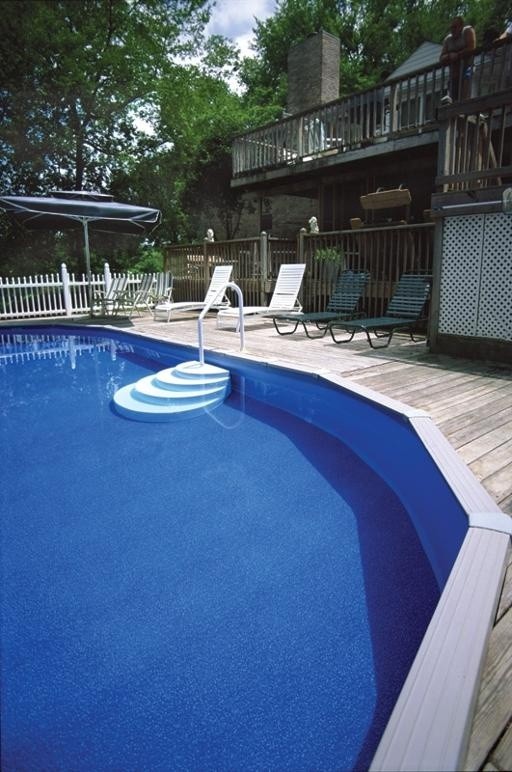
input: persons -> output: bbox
[493,22,512,42]
[439,17,476,104]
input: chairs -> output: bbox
[91,273,133,321]
[214,261,308,332]
[119,272,164,322]
[152,263,232,325]
[263,270,369,340]
[327,274,434,349]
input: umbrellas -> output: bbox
[0,189,162,318]
